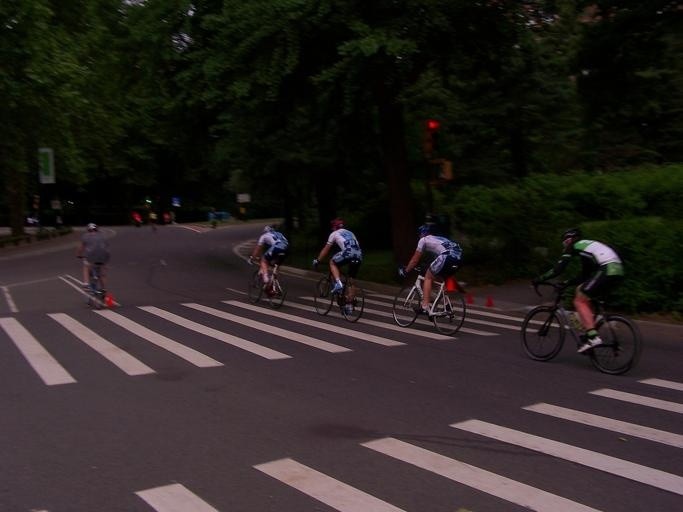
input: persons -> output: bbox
[312,217,364,315]
[248,225,290,292]
[529,227,623,354]
[400,224,463,314]
[77,224,109,290]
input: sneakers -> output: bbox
[82,285,92,291]
[441,304,453,312]
[263,278,269,289]
[341,307,353,315]
[331,282,343,293]
[413,305,429,316]
[577,336,603,353]
[592,314,604,325]
[95,289,107,294]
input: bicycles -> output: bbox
[245,255,287,309]
[520,280,642,375]
[393,266,466,336]
[312,259,364,324]
[77,256,109,309]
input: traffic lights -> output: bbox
[423,120,440,155]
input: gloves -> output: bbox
[313,259,317,265]
[398,267,405,277]
[556,279,569,289]
[248,256,253,265]
[533,277,540,286]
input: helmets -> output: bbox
[88,223,96,230]
[561,229,575,253]
[419,225,429,236]
[264,224,272,232]
[332,219,345,231]
